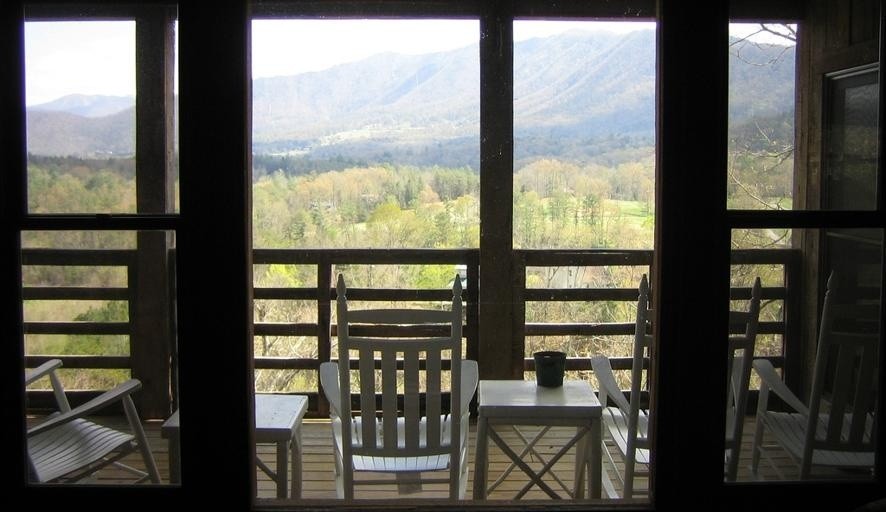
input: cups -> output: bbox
[534,352,566,388]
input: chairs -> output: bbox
[754,269,880,482]
[26,358,163,484]
[591,274,762,500]
[319,274,479,500]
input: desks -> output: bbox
[161,394,308,498]
[473,380,603,499]
